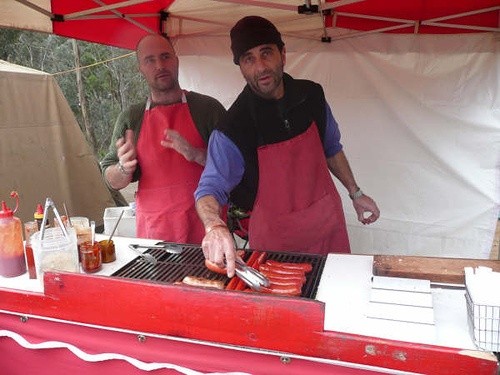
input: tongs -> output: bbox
[222,257,270,291]
[39,197,69,240]
[128,243,183,266]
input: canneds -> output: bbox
[80,239,116,274]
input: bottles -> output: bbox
[67,217,92,264]
[34,203,50,231]
[79,240,103,274]
[22,219,39,279]
[0,192,26,278]
[53,215,67,227]
[99,239,116,263]
[125,202,137,216]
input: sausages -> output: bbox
[174,250,312,298]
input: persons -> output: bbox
[101,34,227,245]
[193,15,380,278]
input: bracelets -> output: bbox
[119,164,128,175]
[350,188,362,200]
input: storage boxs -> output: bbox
[102,207,136,237]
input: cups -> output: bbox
[31,226,80,290]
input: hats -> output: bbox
[230,16,284,65]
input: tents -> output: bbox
[0,0,500,259]
